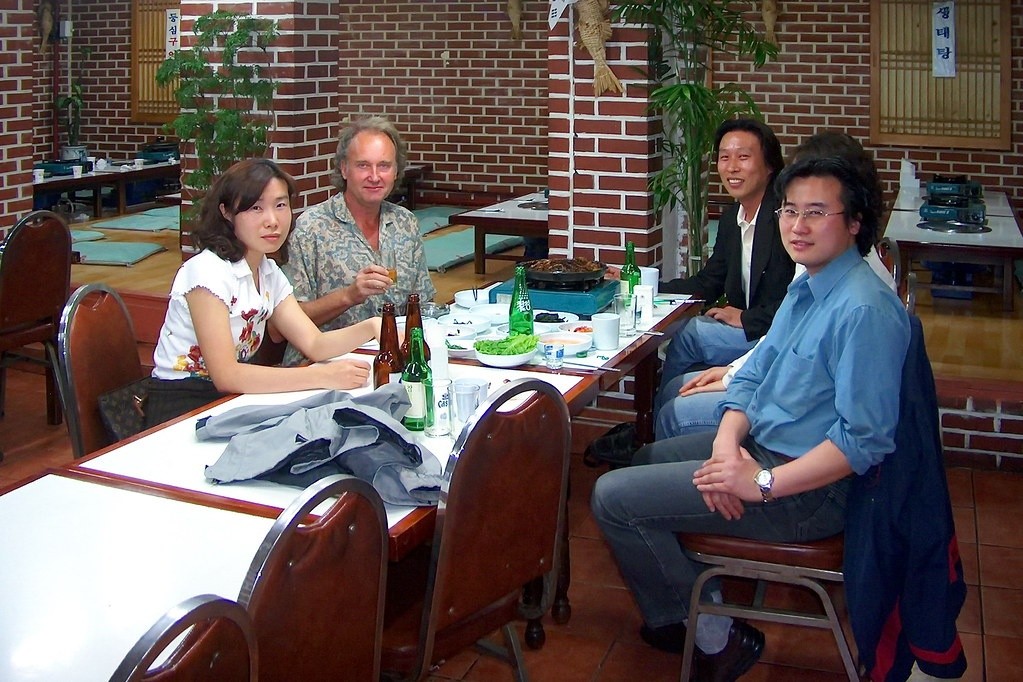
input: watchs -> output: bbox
[755,467,773,504]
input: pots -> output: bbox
[141,137,178,152]
[517,259,608,280]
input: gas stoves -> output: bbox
[135,148,180,161]
[33,160,93,176]
[920,173,987,225]
[489,277,621,321]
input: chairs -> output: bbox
[0,212,571,682]
[680,238,969,682]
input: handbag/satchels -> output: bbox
[99,376,151,444]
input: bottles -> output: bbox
[401,293,432,370]
[509,267,533,337]
[373,302,403,388]
[619,241,640,308]
[402,327,434,431]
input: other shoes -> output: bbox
[590,427,656,464]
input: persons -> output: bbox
[264,116,439,370]
[142,158,405,432]
[587,117,913,682]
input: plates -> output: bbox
[438,288,593,367]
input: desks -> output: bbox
[883,182,1023,311]
[346,280,704,454]
[59,349,600,563]
[0,468,319,682]
[400,162,434,211]
[446,190,548,274]
[31,159,183,218]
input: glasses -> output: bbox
[774,208,845,221]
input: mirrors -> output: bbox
[32,0,182,301]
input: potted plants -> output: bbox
[54,45,91,162]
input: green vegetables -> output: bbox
[474,332,541,354]
[446,341,467,349]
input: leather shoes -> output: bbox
[640,620,687,653]
[689,620,765,682]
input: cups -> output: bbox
[33,169,45,182]
[455,378,487,423]
[641,268,659,296]
[447,384,479,440]
[613,293,637,334]
[87,156,95,171]
[374,249,398,285]
[72,166,82,179]
[134,158,144,170]
[424,378,453,436]
[543,343,564,369]
[591,312,620,351]
